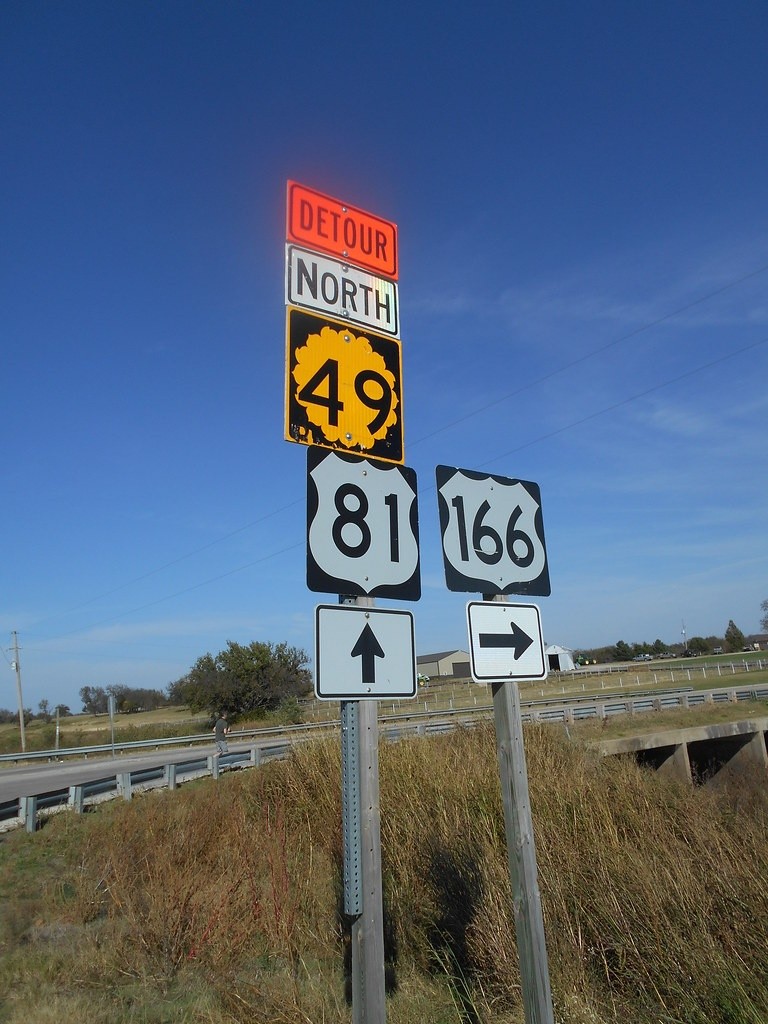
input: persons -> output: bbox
[212,711,231,754]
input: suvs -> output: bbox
[713,647,724,654]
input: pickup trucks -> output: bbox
[657,652,676,658]
[633,654,654,662]
